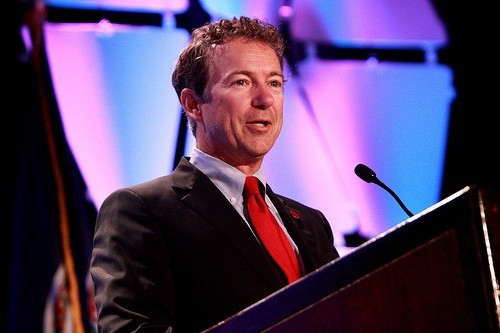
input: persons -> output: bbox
[90,16,341,333]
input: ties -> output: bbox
[246,176,300,284]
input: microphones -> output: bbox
[354,164,414,217]
